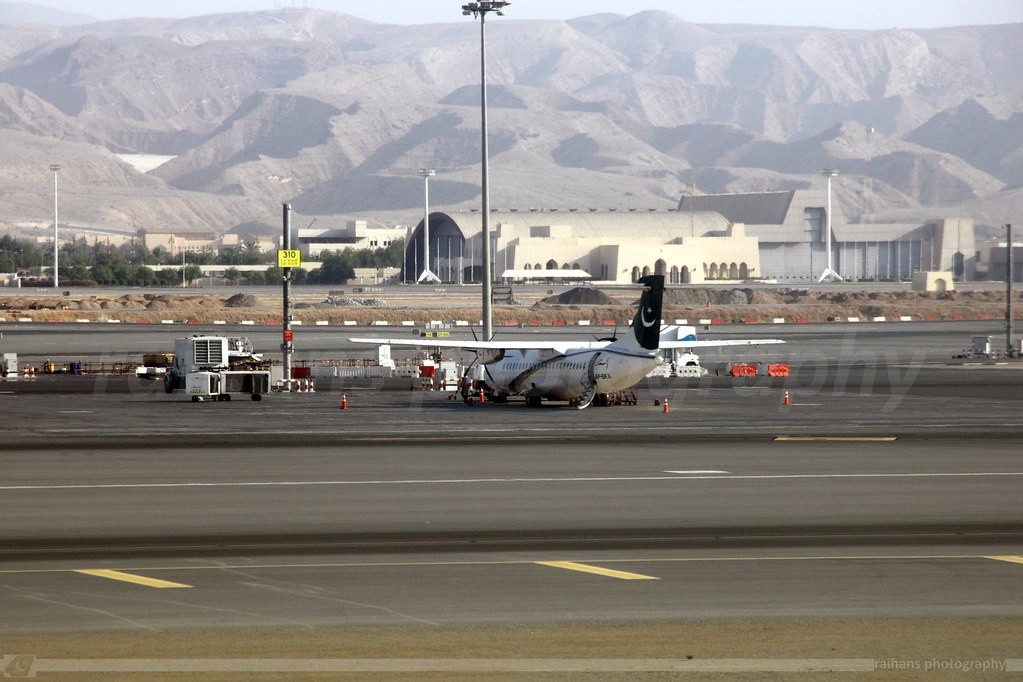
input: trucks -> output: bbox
[162,336,273,402]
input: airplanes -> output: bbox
[342,274,792,412]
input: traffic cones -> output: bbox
[661,397,670,413]
[340,393,348,409]
[479,388,485,404]
[782,390,790,405]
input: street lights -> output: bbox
[462,0,513,341]
[49,164,62,296]
[820,166,844,284]
[417,167,439,284]
